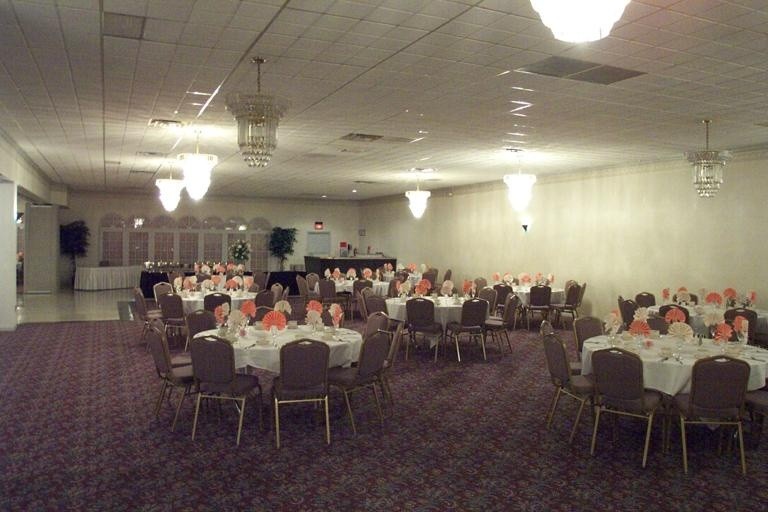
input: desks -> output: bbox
[579,331,767,407]
[193,322,363,398]
[72,265,142,291]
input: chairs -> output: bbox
[589,347,667,469]
[151,263,438,323]
[271,339,332,452]
[330,311,407,440]
[573,315,606,361]
[661,354,752,475]
[545,333,597,444]
[442,261,593,364]
[540,320,581,421]
[146,326,196,436]
[134,287,192,354]
[356,285,441,366]
[189,334,266,450]
[612,290,764,343]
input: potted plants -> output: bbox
[59,220,92,288]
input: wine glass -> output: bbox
[605,325,748,366]
[399,289,476,306]
[215,312,343,353]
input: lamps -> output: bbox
[503,155,538,213]
[155,166,185,212]
[223,55,292,170]
[527,0,631,42]
[177,129,218,201]
[404,178,431,219]
[683,118,734,200]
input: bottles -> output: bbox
[142,261,180,274]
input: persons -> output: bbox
[16,251,24,287]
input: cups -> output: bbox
[180,288,243,298]
